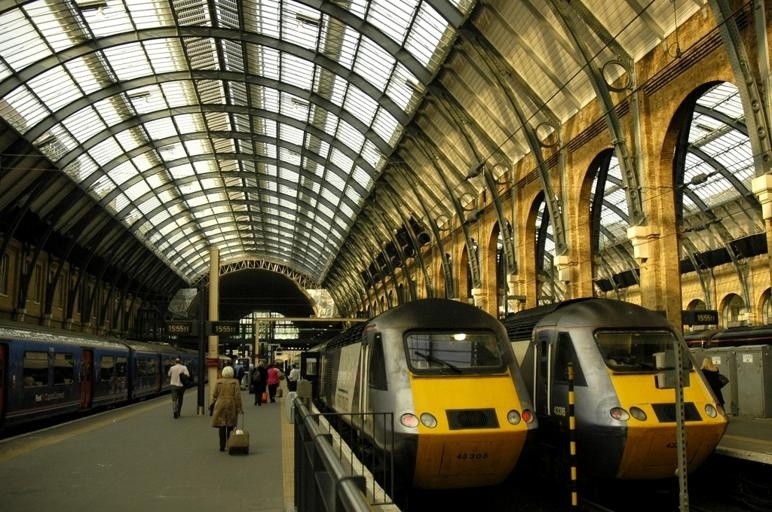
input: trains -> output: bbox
[0,323,230,439]
[501,299,730,481]
[284,297,541,490]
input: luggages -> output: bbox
[262,392,269,403]
[228,412,249,455]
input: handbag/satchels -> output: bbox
[180,373,194,388]
[209,402,214,416]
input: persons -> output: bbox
[700,357,727,415]
[210,365,244,451]
[235,363,245,386]
[249,357,299,406]
[167,358,191,419]
